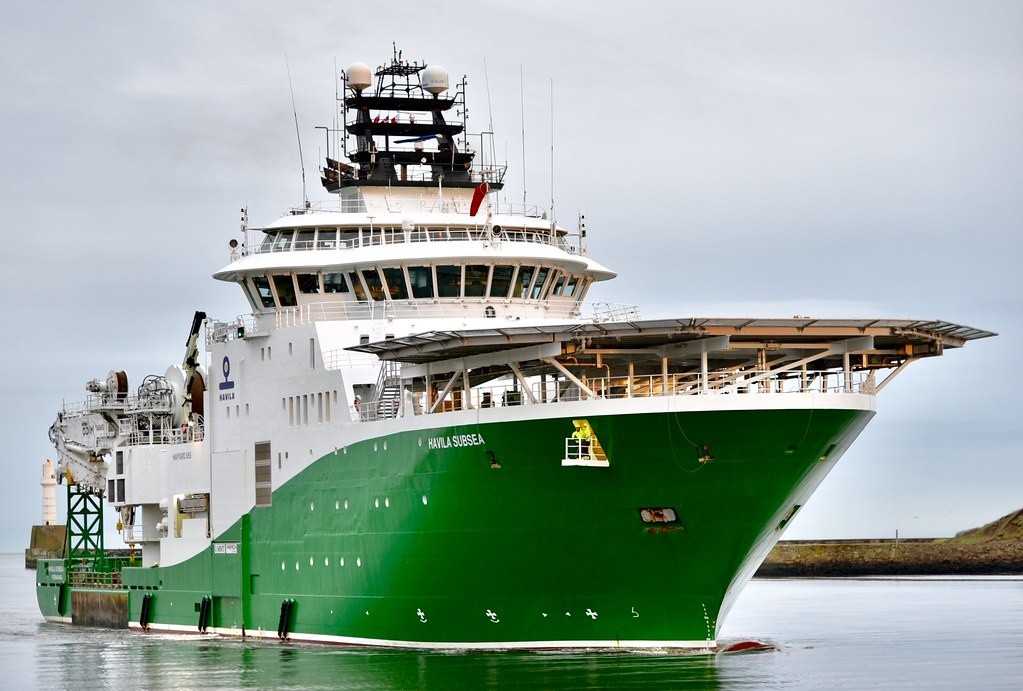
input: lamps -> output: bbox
[422,65,450,100]
[345,63,372,97]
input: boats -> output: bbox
[26,41,1001,661]
